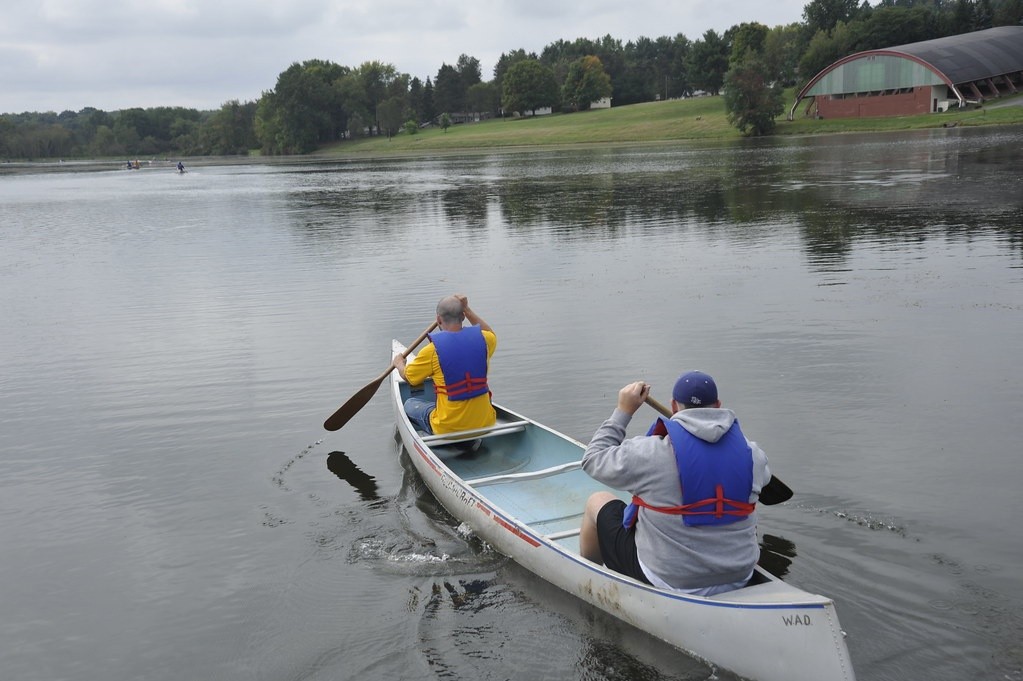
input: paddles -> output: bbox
[322,319,439,432]
[639,384,795,506]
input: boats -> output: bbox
[388,338,857,680]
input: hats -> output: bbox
[673,370,718,406]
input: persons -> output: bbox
[177,162,184,171]
[579,370,771,597]
[134,159,139,165]
[126,160,131,166]
[393,295,497,451]
